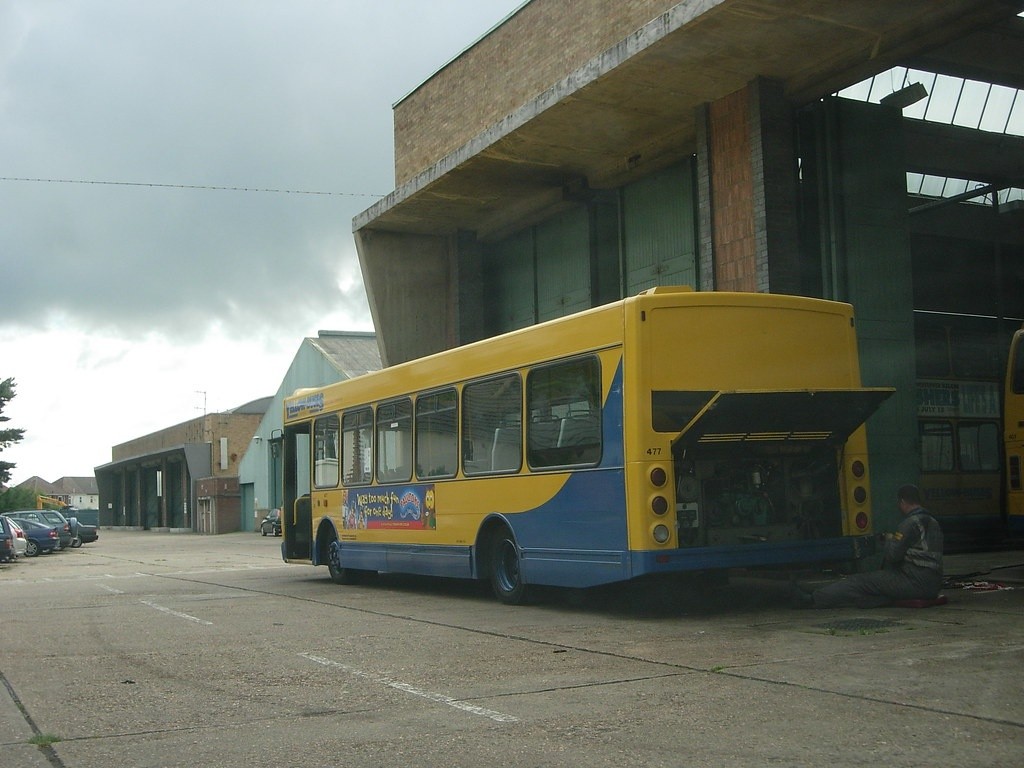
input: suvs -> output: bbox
[260,507,281,536]
[2,511,77,552]
[0,517,27,561]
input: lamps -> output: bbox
[253,436,262,441]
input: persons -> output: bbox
[785,484,944,609]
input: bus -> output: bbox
[1004,329,1024,519]
[269,286,897,606]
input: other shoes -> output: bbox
[790,586,814,610]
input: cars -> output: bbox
[12,518,60,557]
[65,518,98,548]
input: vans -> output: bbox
[0,516,13,561]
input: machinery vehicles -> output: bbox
[36,495,78,511]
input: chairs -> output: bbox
[453,408,601,474]
